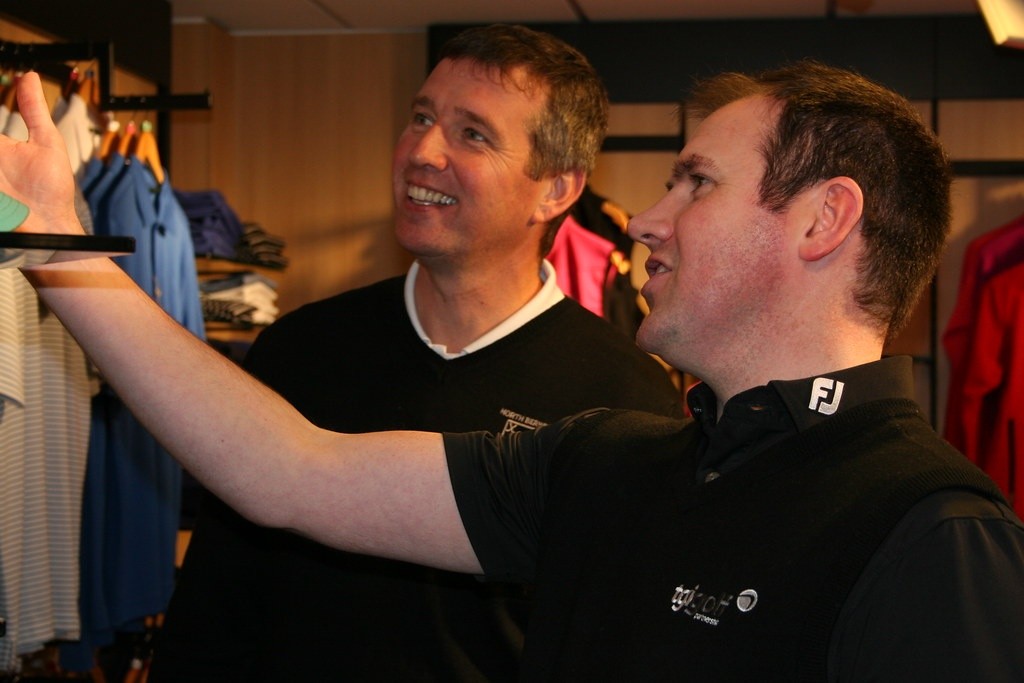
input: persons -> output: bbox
[148,28,682,683]
[0,62,1024,683]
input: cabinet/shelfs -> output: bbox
[194,257,280,345]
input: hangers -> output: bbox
[0,40,168,189]
